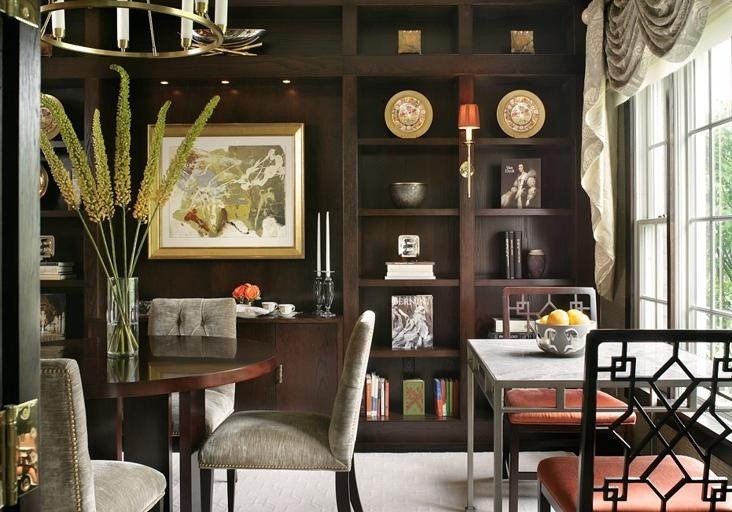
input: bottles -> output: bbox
[527,249,546,278]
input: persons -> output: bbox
[512,163,536,209]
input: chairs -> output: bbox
[494,282,637,512]
[532,328,732,511]
[40,297,376,512]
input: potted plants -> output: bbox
[30,62,224,361]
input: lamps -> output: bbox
[39,0,263,59]
[454,103,480,199]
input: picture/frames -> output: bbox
[145,120,306,260]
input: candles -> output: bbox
[325,211,331,278]
[316,212,322,276]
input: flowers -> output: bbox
[232,283,262,302]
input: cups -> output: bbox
[278,304,295,314]
[262,302,278,311]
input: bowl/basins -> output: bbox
[389,181,428,209]
[535,318,597,357]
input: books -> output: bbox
[403,415,425,422]
[402,378,425,416]
[500,231,510,279]
[436,417,455,420]
[514,231,522,279]
[40,261,77,281]
[509,231,515,279]
[367,416,389,421]
[434,377,459,417]
[365,371,390,417]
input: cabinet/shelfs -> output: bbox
[38,0,603,451]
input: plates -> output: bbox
[40,165,49,199]
[384,89,434,138]
[276,311,299,314]
[496,89,546,138]
[40,95,65,140]
